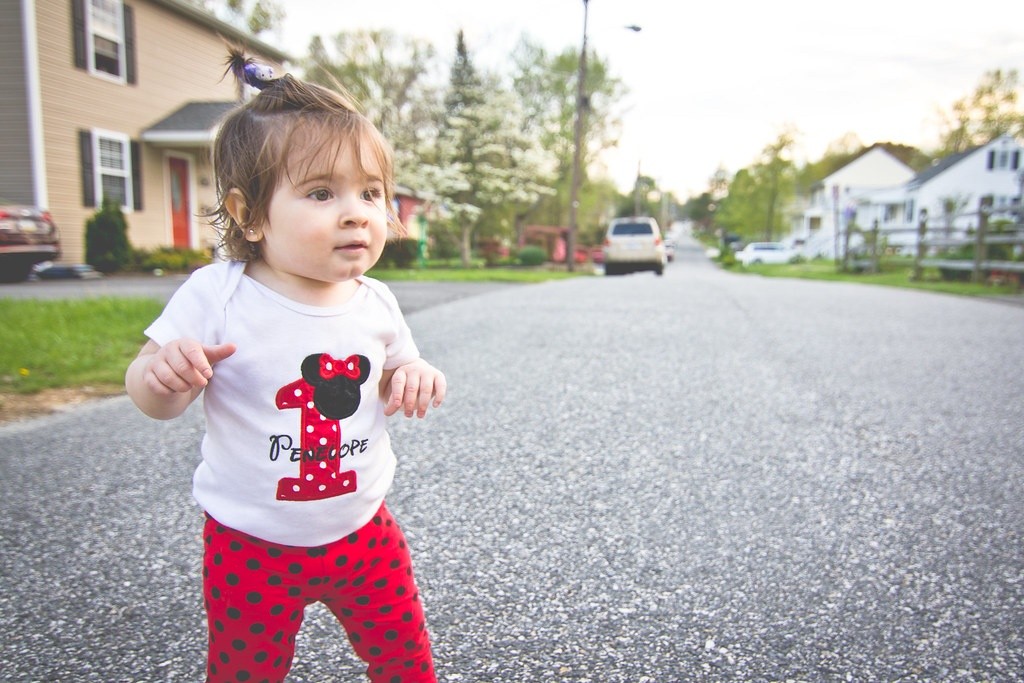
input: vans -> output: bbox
[600,217,669,276]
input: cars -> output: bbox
[736,242,796,267]
[0,198,61,282]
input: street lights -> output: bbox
[564,0,641,271]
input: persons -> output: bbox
[123,47,446,683]
